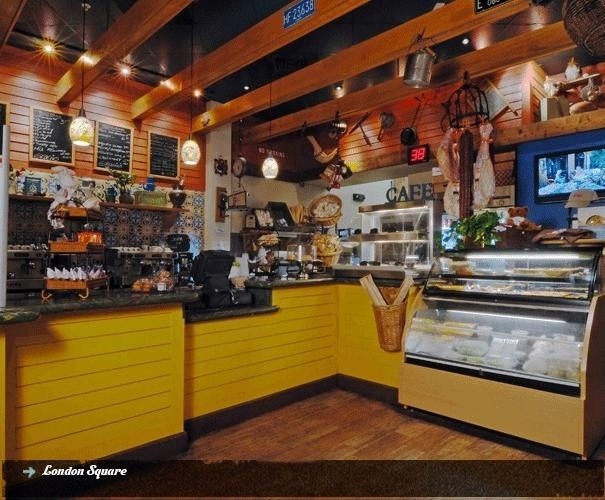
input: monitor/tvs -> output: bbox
[533,144,605,205]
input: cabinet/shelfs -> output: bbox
[396,241,604,463]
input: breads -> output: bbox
[131,270,174,294]
[585,215,605,226]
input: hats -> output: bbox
[562,188,598,209]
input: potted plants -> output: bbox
[108,166,136,204]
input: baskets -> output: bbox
[318,247,343,267]
[307,194,343,226]
[371,297,407,354]
[60,207,102,218]
[43,275,109,291]
[48,240,105,254]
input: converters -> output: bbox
[190,250,235,282]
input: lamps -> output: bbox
[67,0,96,149]
[180,22,200,167]
[260,55,279,181]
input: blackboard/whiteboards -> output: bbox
[0,101,180,182]
